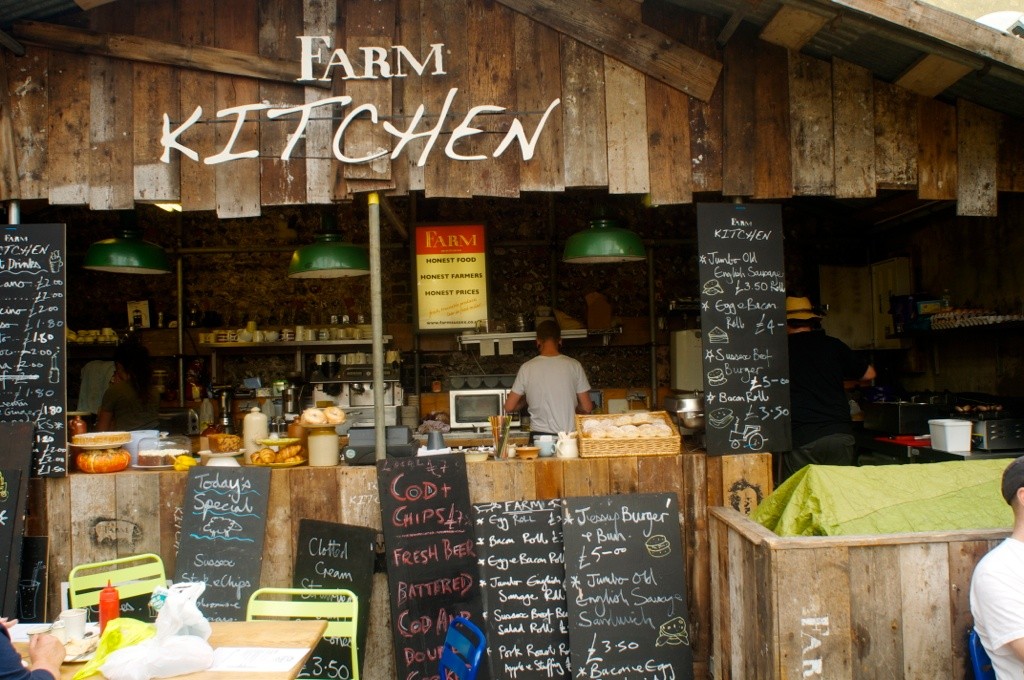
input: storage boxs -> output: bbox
[929,418,973,452]
[575,410,681,458]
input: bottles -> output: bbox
[242,406,269,463]
[941,288,952,311]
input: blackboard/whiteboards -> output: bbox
[172,465,273,623]
[469,497,574,679]
[560,491,697,679]
[377,451,491,680]
[289,518,374,680]
[0,223,72,476]
[696,202,794,458]
[68,585,160,624]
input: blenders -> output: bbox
[216,388,234,434]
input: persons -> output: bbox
[0,614,65,680]
[967,455,1024,679]
[503,320,593,446]
[92,337,162,432]
[783,296,879,475]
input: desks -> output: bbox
[6,621,326,680]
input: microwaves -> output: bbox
[448,388,521,429]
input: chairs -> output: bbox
[245,588,359,680]
[68,554,166,623]
[437,616,486,680]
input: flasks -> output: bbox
[283,389,298,414]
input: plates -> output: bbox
[68,440,133,450]
[132,464,175,471]
[61,626,98,663]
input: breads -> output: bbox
[251,445,307,464]
[208,434,241,453]
[584,412,673,439]
[78,450,132,472]
[301,406,345,425]
[72,431,132,446]
[139,449,191,466]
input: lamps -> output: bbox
[80,229,175,275]
[287,232,371,280]
[561,218,647,265]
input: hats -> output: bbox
[785,296,825,322]
[1000,456,1024,504]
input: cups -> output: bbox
[59,608,87,641]
[556,438,579,459]
[192,386,204,402]
[28,628,52,640]
[534,439,557,456]
[66,327,118,344]
[198,321,398,366]
[427,430,445,452]
[491,425,509,461]
